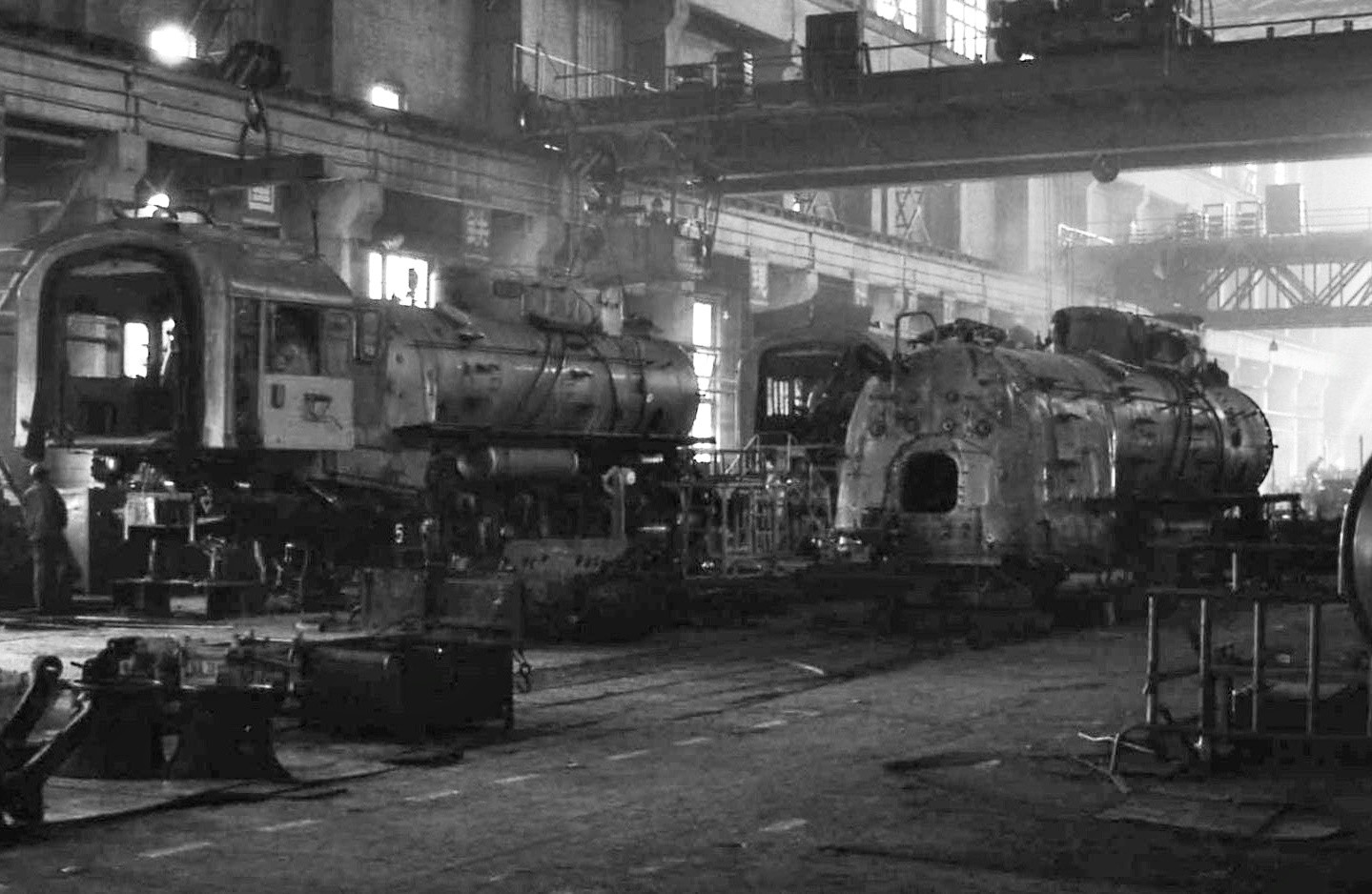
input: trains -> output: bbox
[290,267,1313,656]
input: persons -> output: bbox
[424,494,475,567]
[22,463,75,615]
[1306,457,1323,484]
